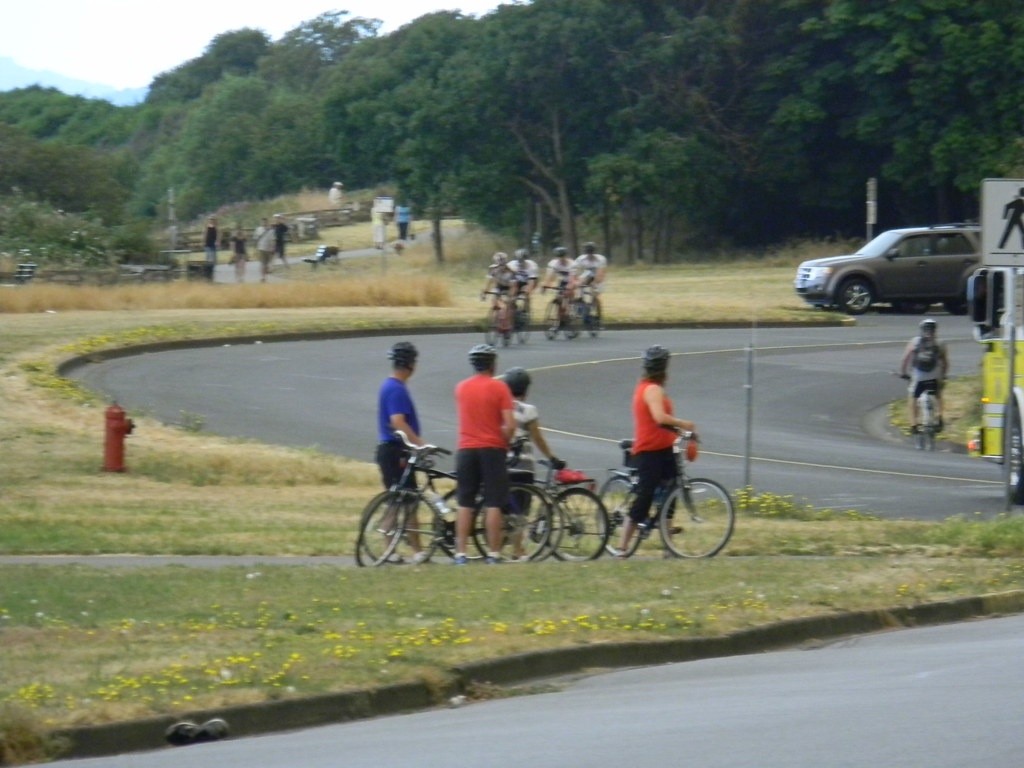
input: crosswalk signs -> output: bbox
[982,178,1024,266]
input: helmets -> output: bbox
[920,318,936,327]
[554,247,566,257]
[515,249,528,259]
[468,343,496,356]
[389,342,417,356]
[584,242,598,252]
[644,345,669,361]
[507,367,530,385]
[493,252,508,261]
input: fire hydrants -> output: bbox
[102,400,135,472]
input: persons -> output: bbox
[619,345,695,558]
[204,218,219,260]
[271,214,289,266]
[455,343,566,563]
[328,181,344,204]
[901,319,947,434]
[370,207,394,249]
[252,218,276,281]
[399,202,412,238]
[481,242,608,344]
[223,231,250,264]
[376,341,433,564]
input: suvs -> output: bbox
[792,223,982,314]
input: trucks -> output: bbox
[966,264,1024,507]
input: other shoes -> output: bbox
[485,556,503,564]
[911,427,919,435]
[387,553,403,563]
[934,421,943,433]
[413,551,430,561]
[455,556,467,565]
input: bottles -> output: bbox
[426,490,452,514]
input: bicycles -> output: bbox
[595,422,735,561]
[355,430,612,568]
[480,284,604,349]
[891,372,945,450]
